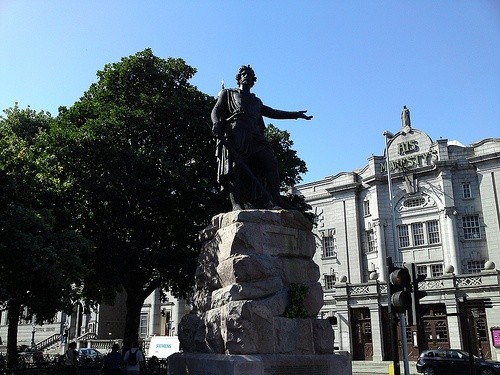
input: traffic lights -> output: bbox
[389,268,412,312]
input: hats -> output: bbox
[133,341,141,348]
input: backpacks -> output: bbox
[127,349,138,366]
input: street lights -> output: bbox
[383,130,410,375]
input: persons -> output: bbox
[210,63,314,212]
[58,326,69,344]
[400,105,410,128]
[168,324,175,336]
[61,341,146,375]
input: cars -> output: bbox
[417,349,500,375]
[78,348,103,358]
